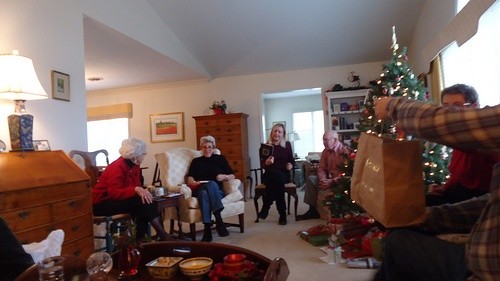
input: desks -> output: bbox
[153,191,183,238]
[72,241,289,281]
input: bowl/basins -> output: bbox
[179,257,213,276]
[146,256,183,279]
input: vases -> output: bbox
[213,107,224,115]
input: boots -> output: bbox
[151,217,175,241]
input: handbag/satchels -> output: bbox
[349,115,426,227]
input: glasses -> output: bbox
[443,102,471,108]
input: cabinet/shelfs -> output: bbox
[193,113,251,203]
[325,90,371,142]
[0,149,95,261]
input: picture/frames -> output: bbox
[51,69,71,101]
[32,140,51,152]
[150,112,185,142]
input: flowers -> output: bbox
[208,100,227,111]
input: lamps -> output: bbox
[348,72,361,88]
[288,132,302,159]
[0,50,49,152]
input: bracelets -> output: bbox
[226,175,228,180]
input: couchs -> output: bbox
[303,151,322,180]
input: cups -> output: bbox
[155,188,164,197]
[224,254,248,279]
[38,256,65,281]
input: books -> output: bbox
[332,117,348,130]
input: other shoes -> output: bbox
[297,206,320,219]
[279,213,286,225]
[201,233,213,242]
[258,204,271,219]
[215,218,230,237]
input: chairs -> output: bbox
[70,148,134,250]
[155,147,245,242]
[249,165,299,223]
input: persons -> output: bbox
[425,83,491,234]
[296,130,353,221]
[184,136,235,242]
[258,124,295,225]
[371,97,500,281]
[92,137,178,241]
